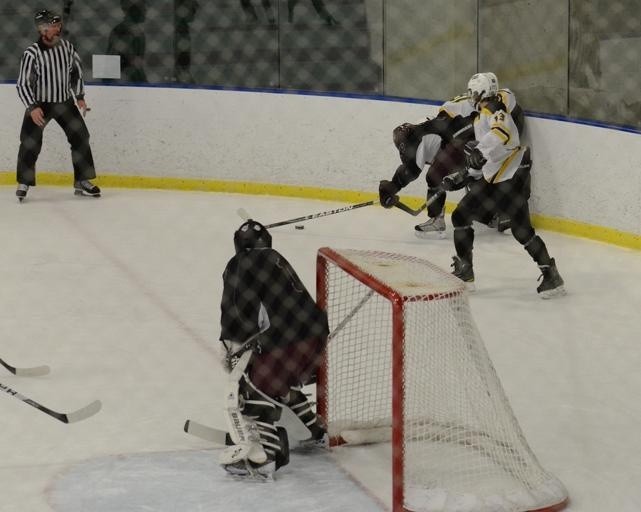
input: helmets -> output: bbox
[466,71,499,111]
[232,217,273,254]
[392,122,412,151]
[34,9,59,26]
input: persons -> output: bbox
[441,71,564,294]
[379,87,525,233]
[15,9,101,199]
[219,221,331,477]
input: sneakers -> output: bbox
[413,215,447,232]
[73,178,101,197]
[297,431,330,449]
[15,183,29,199]
[222,455,277,476]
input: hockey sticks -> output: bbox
[235,198,378,228]
[0,357,50,377]
[392,189,448,216]
[1,385,102,424]
[184,343,234,448]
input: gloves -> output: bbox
[378,181,398,208]
[441,168,474,191]
[465,147,486,171]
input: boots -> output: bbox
[537,257,564,293]
[450,255,475,283]
